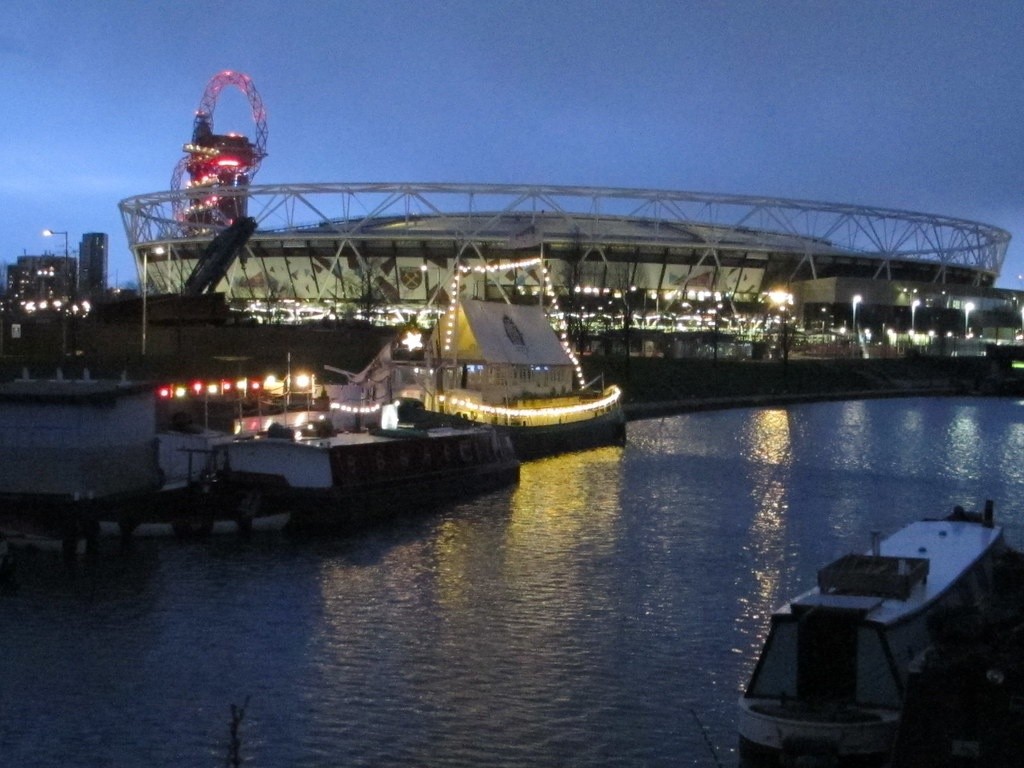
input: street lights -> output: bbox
[43,229,70,297]
[851,294,861,335]
[421,265,441,353]
[139,245,166,354]
[909,300,920,337]
[964,302,974,339]
[771,291,791,365]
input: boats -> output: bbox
[191,396,520,520]
[388,256,629,449]
[735,499,1024,768]
